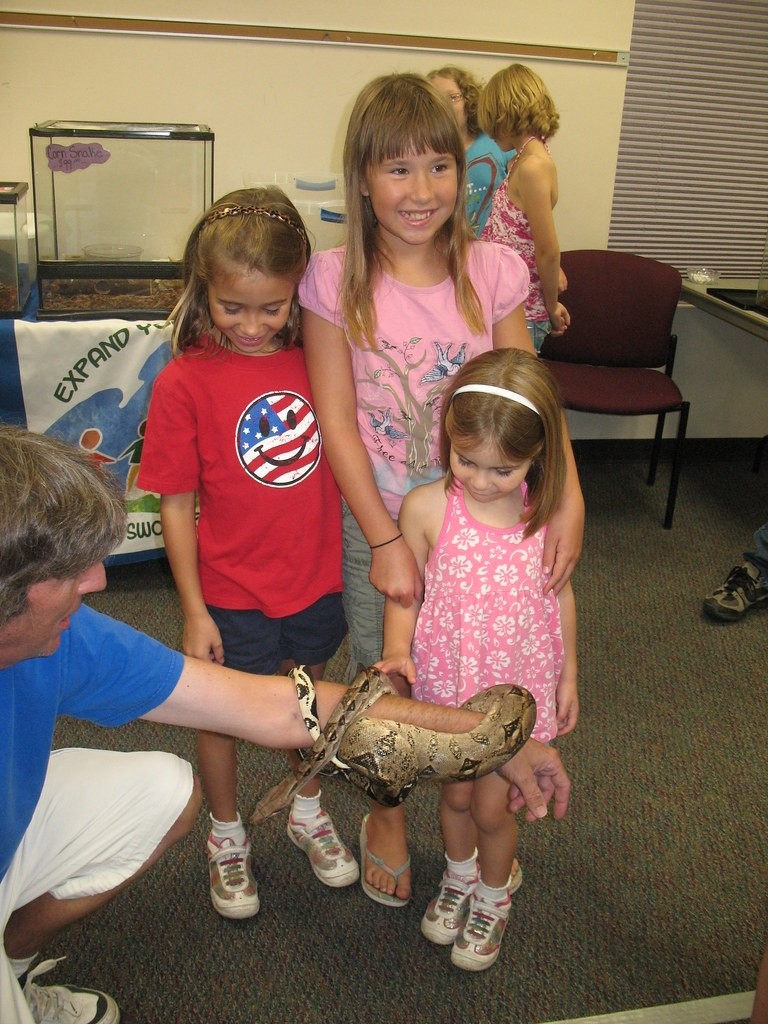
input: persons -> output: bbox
[135,186,361,919]
[426,66,567,297]
[298,73,585,907]
[477,64,570,354]
[0,421,571,1024]
[703,522,768,620]
[372,348,579,972]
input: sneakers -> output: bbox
[206,830,259,918]
[287,804,361,887]
[17,955,120,1024]
[704,562,768,623]
[420,868,480,945]
[450,886,512,972]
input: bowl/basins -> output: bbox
[687,266,721,285]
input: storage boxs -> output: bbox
[0,121,215,321]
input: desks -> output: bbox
[681,276,768,343]
[0,276,200,563]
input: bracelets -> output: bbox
[370,533,403,549]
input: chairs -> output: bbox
[536,248,691,529]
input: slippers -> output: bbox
[508,865,523,894]
[359,815,412,906]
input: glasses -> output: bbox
[447,93,465,104]
